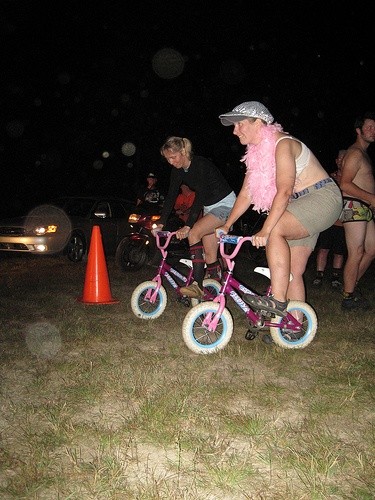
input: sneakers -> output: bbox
[354,282,369,308]
[180,280,204,299]
[341,291,373,312]
[331,270,343,289]
[311,271,324,288]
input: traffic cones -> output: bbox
[79,225,120,305]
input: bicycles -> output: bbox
[182,232,318,356]
[131,230,223,321]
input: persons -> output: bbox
[338,116,375,309]
[151,136,237,299]
[215,101,343,343]
[313,150,347,288]
[135,169,166,206]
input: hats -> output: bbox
[219,101,274,126]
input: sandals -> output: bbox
[243,293,290,316]
[263,328,303,343]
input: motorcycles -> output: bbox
[113,211,191,274]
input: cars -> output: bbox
[0,192,132,264]
